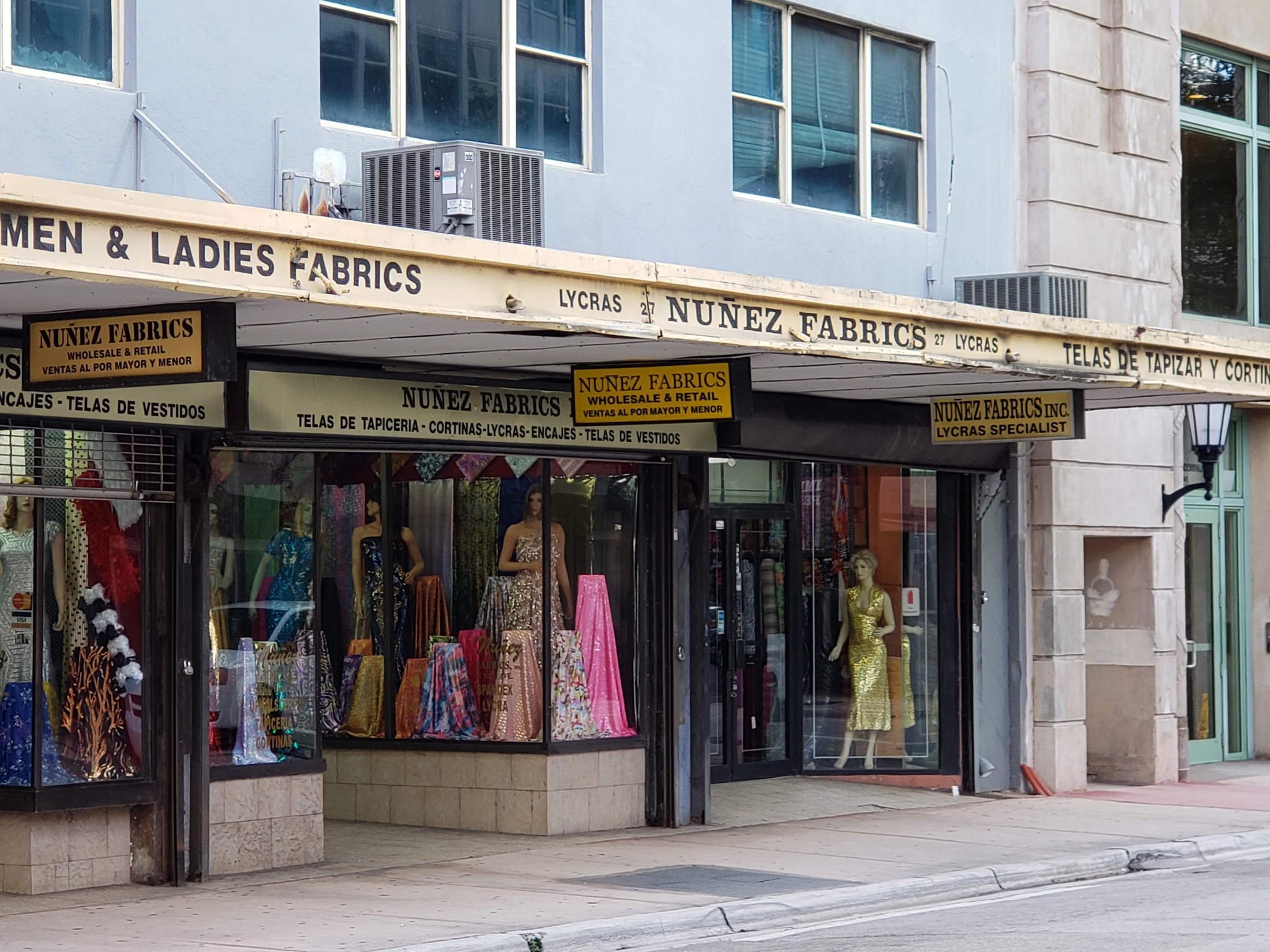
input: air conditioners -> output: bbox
[954,271,1089,318]
[361,140,545,249]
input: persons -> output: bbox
[828,549,895,768]
[250,494,326,646]
[351,486,425,681]
[208,496,235,597]
[499,484,574,670]
[0,477,67,690]
[902,624,923,728]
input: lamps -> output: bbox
[1162,401,1233,524]
